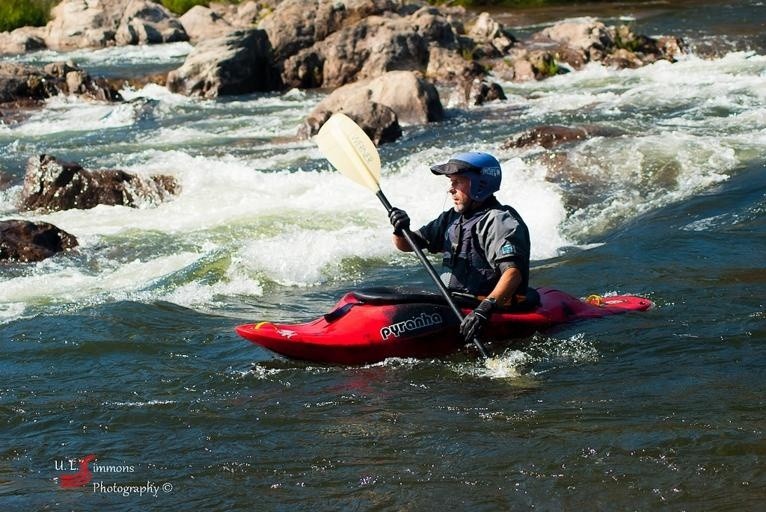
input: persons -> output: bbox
[385,151,531,342]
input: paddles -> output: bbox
[316,111,540,389]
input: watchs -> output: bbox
[483,296,497,305]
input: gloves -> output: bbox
[458,295,497,345]
[386,204,413,238]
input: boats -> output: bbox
[234,287,652,366]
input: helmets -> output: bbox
[446,151,504,202]
